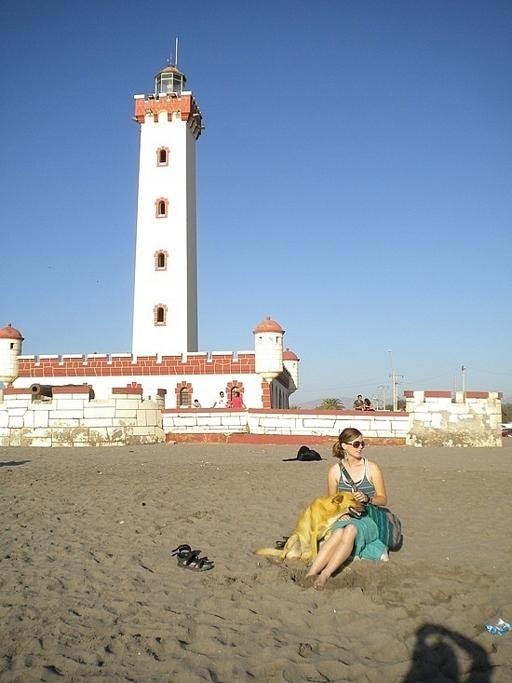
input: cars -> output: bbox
[502,428,512,438]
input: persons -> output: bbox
[352,394,365,410]
[193,398,201,408]
[360,398,376,411]
[228,391,248,409]
[304,426,388,591]
[209,390,230,409]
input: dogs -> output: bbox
[283,446,321,461]
[253,491,367,568]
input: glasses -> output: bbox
[344,440,365,448]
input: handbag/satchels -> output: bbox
[368,504,403,551]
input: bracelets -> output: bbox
[364,493,370,504]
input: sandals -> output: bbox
[172,543,214,572]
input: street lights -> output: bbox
[376,383,386,411]
[386,349,396,412]
